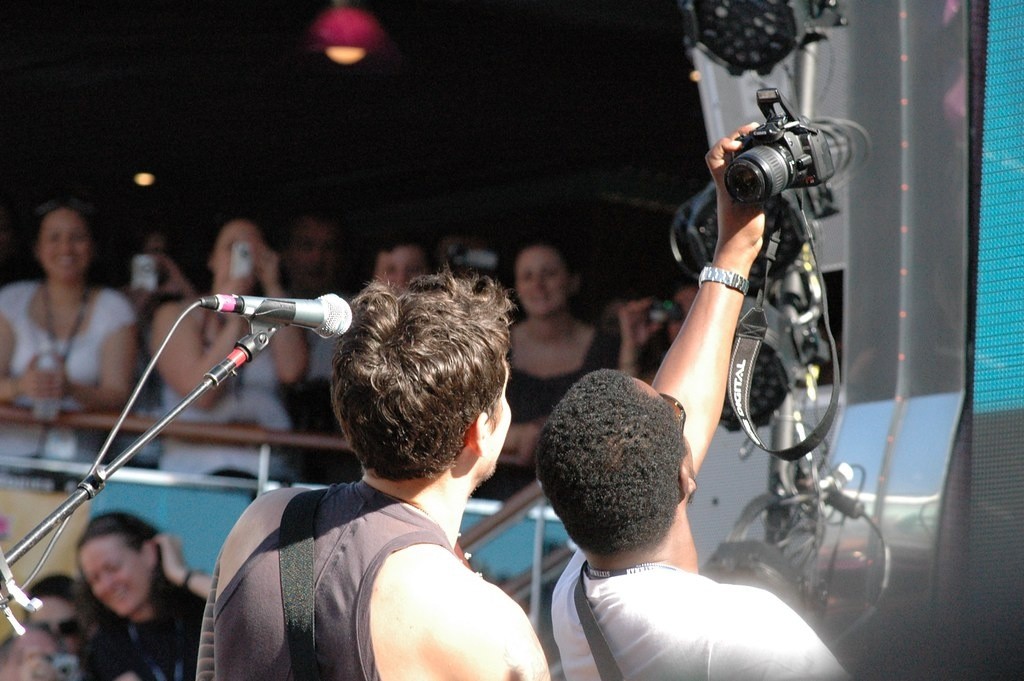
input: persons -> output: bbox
[0,206,700,681]
[540,122,853,681]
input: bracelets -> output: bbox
[181,570,192,590]
[699,265,750,294]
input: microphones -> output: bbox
[199,294,352,339]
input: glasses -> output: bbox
[659,391,686,437]
[34,619,80,639]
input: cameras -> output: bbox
[642,301,683,326]
[448,243,498,272]
[132,254,159,292]
[724,87,835,207]
